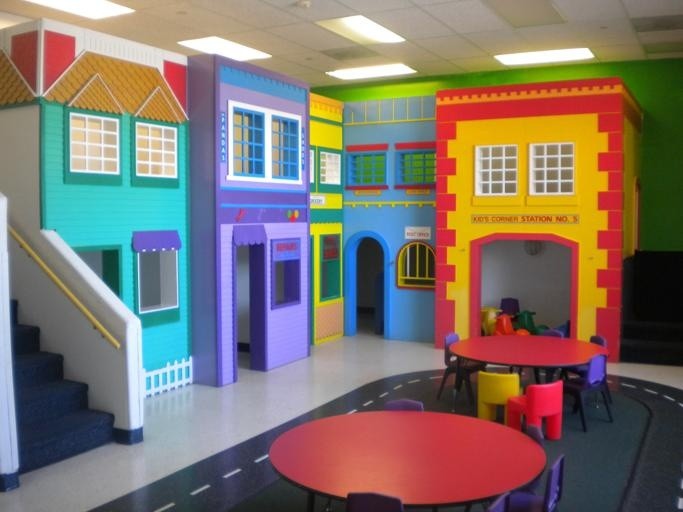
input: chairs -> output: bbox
[503,455,580,506]
[503,381,572,438]
[573,352,611,433]
[381,393,429,407]
[339,485,405,506]
[467,368,523,418]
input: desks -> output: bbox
[257,415,553,506]
[427,338,606,399]
